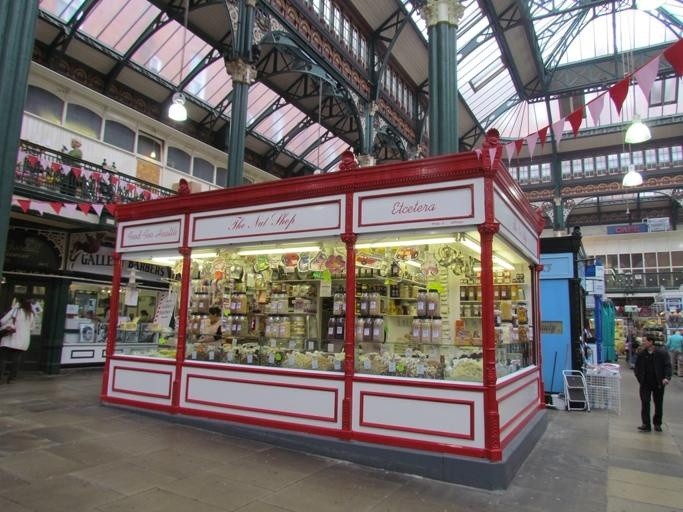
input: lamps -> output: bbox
[622,1,652,189]
[169,0,189,121]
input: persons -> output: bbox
[0,293,34,384]
[97,323,108,343]
[104,306,111,322]
[635,333,672,432]
[123,310,153,331]
[61,137,82,197]
[666,330,683,376]
[82,326,93,341]
[191,306,225,343]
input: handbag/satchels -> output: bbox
[0,307,17,336]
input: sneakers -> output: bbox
[638,424,662,432]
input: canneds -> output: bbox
[460,305,482,317]
[460,285,524,300]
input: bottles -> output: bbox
[189,267,529,346]
